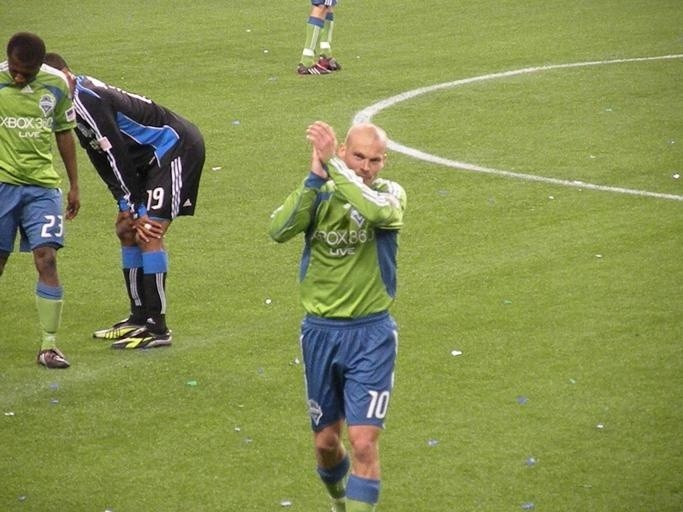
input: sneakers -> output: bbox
[297,54,341,75]
[37,348,70,368]
[91,319,172,349]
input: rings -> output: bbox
[144,222,153,232]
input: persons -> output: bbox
[0,31,82,370]
[41,53,207,351]
[265,118,410,512]
[295,1,343,77]
